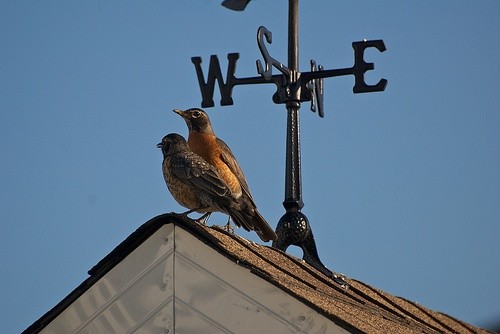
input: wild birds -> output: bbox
[156,133,255,234]
[173,107,279,242]
